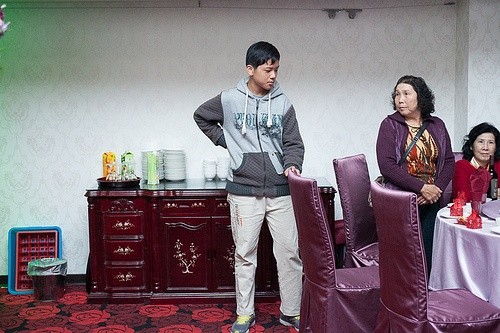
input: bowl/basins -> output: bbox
[203,151,231,181]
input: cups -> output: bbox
[469,192,486,204]
[105,162,137,181]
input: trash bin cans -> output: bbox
[26,257,68,301]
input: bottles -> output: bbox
[486,154,499,200]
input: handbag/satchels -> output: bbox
[368,176,386,207]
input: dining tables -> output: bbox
[428,197,500,311]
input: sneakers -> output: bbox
[279,312,309,332]
[231,312,256,332]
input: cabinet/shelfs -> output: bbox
[274,185,338,301]
[84,189,150,303]
[146,188,276,305]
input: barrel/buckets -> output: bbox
[27,258,68,300]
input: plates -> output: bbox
[490,226,500,235]
[440,212,471,218]
[141,149,186,182]
[447,202,471,208]
[96,176,142,189]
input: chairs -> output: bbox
[372,180,500,333]
[287,168,380,333]
[334,153,380,268]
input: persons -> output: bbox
[194,40,307,332]
[376,74,456,274]
[450,122,499,207]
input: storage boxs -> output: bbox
[7,226,63,294]
